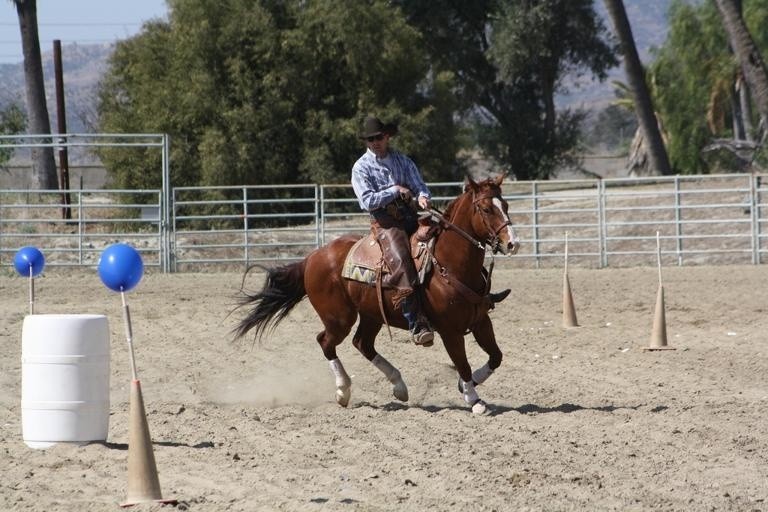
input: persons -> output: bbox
[351,118,434,344]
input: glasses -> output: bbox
[368,135,384,142]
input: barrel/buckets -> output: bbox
[21,313,110,449]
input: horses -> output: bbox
[225,171,521,418]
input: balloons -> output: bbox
[14,247,44,277]
[97,243,143,292]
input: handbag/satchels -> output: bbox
[386,196,417,221]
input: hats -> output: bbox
[359,118,397,138]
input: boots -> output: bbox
[490,289,511,302]
[402,295,434,347]
